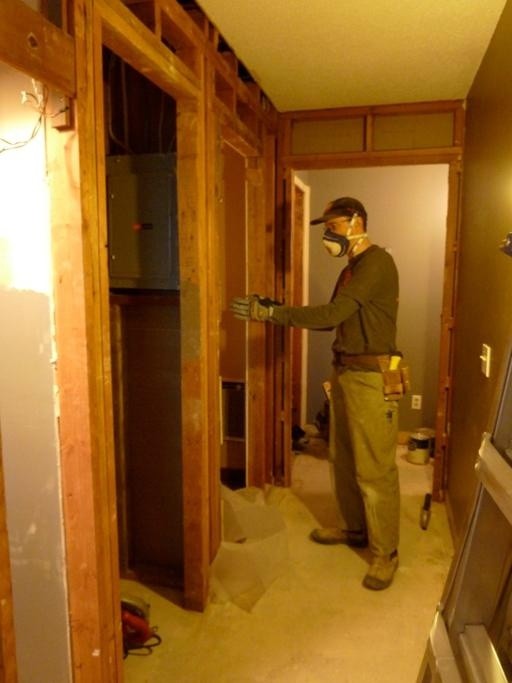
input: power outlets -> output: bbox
[411,394,422,410]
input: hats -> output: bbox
[309,198,368,226]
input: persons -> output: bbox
[230,196,401,590]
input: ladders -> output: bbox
[415,329,512,683]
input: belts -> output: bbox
[329,348,378,366]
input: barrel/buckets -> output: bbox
[409,428,434,465]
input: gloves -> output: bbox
[229,294,274,323]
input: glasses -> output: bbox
[328,220,350,230]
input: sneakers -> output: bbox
[311,526,368,548]
[362,548,399,590]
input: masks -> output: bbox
[321,212,368,257]
